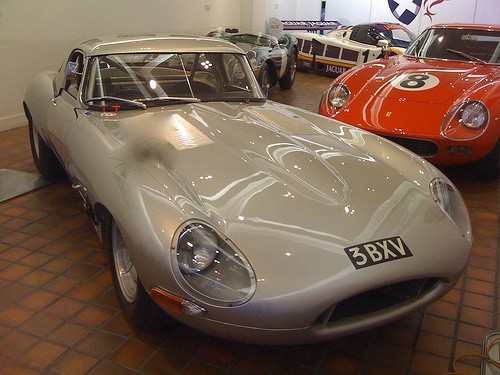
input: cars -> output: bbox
[289,23,416,78]
[207,29,299,98]
[318,24,500,169]
[22,33,471,347]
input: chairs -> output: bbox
[171,80,212,94]
[438,43,472,61]
